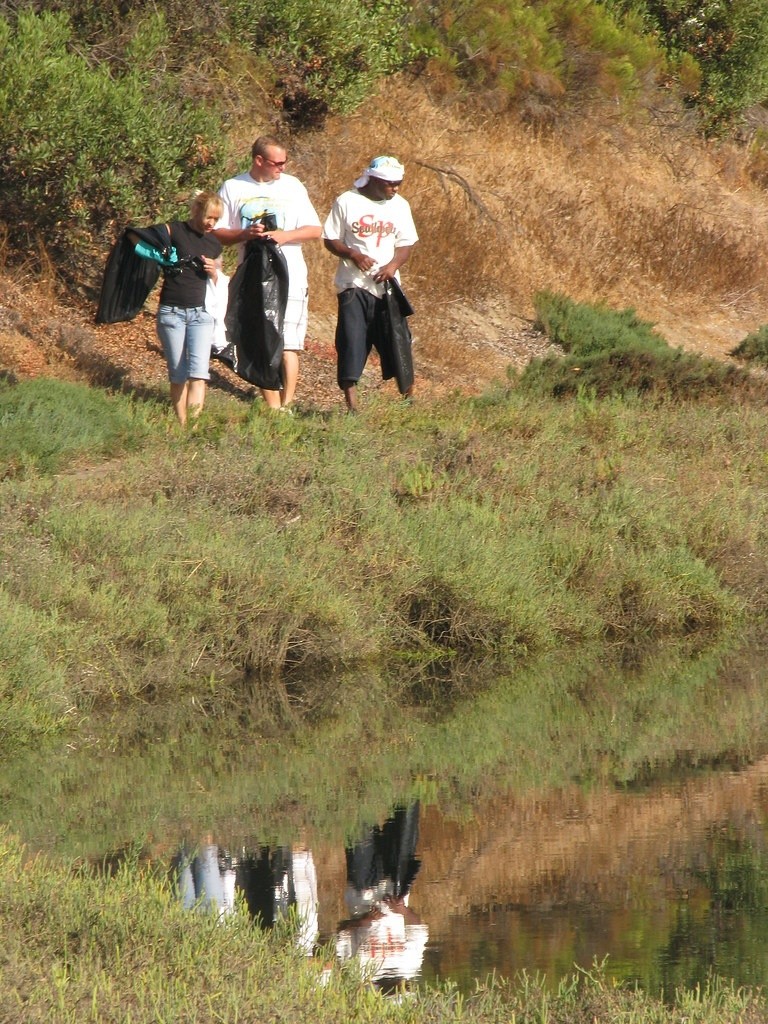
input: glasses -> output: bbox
[261,155,288,167]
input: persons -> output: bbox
[176,825,318,968]
[335,801,430,1006]
[211,133,323,413]
[128,190,224,431]
[320,156,420,411]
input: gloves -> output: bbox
[134,239,178,266]
